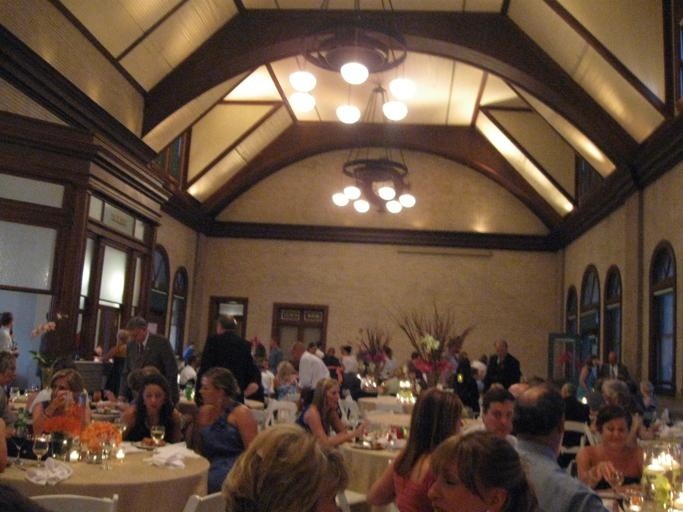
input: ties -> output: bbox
[138,343,144,361]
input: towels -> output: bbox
[142,440,202,472]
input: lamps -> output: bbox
[332,84,417,214]
[289,0,416,124]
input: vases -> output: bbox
[39,368,53,391]
[365,373,379,391]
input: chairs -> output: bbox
[334,482,368,512]
[29,494,119,512]
[560,420,587,453]
[183,491,226,512]
[261,400,298,428]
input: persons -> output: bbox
[0,313,394,512]
[368,338,665,512]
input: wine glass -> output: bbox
[10,387,20,408]
[33,435,48,471]
[621,488,644,512]
[13,425,26,465]
[25,388,33,400]
[150,425,164,452]
[609,461,624,496]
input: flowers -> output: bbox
[395,297,457,387]
[26,313,69,366]
[355,326,393,372]
